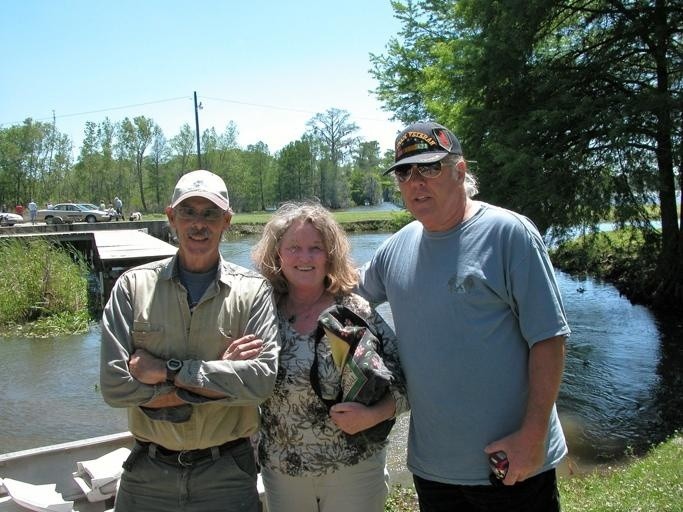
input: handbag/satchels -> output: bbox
[316,305,396,442]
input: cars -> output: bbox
[35,203,110,224]
[78,204,118,220]
[1,211,22,227]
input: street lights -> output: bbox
[48,109,55,175]
[192,91,204,171]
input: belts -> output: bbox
[135,439,246,469]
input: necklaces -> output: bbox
[280,289,324,326]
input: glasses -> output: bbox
[394,161,451,184]
[174,207,228,220]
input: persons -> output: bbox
[98,199,105,210]
[28,199,38,225]
[97,167,281,512]
[219,199,410,511]
[16,201,23,217]
[344,118,569,511]
[113,196,125,222]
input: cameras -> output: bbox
[489,451,510,480]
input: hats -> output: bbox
[383,122,462,175]
[171,170,228,211]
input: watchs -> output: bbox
[166,358,183,383]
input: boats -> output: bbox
[1,426,138,511]
